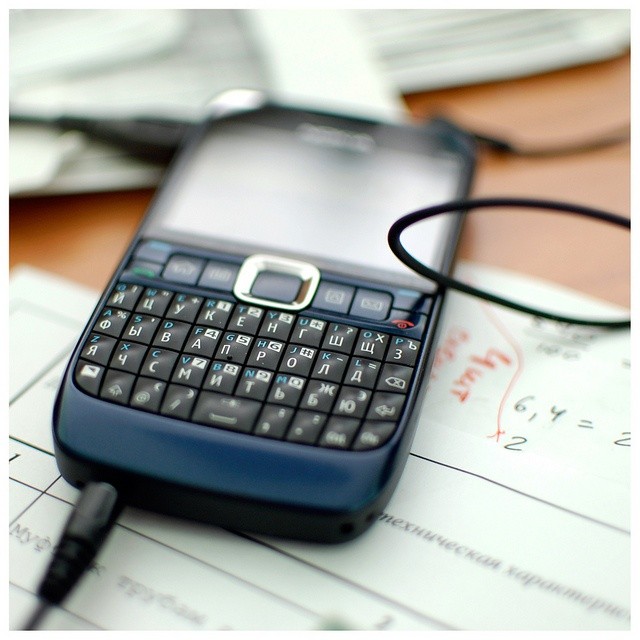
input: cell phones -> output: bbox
[50,94,478,545]
